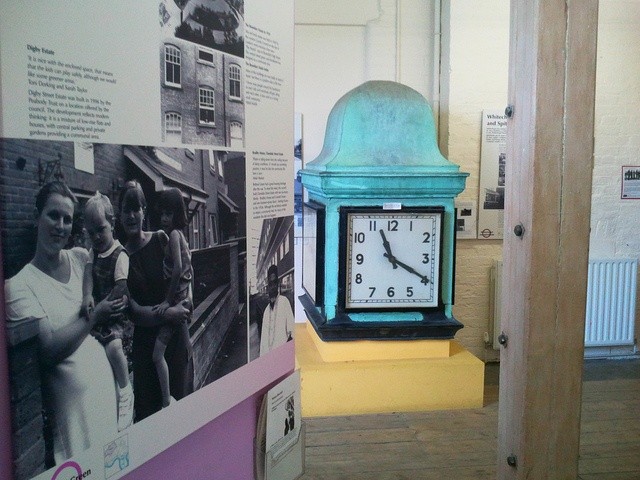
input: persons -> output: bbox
[79,193,135,433]
[3,180,119,465]
[116,180,195,424]
[260,265,295,357]
[152,187,193,411]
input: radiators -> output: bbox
[485,257,639,356]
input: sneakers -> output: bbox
[117,384,136,429]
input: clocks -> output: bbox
[339,206,444,313]
